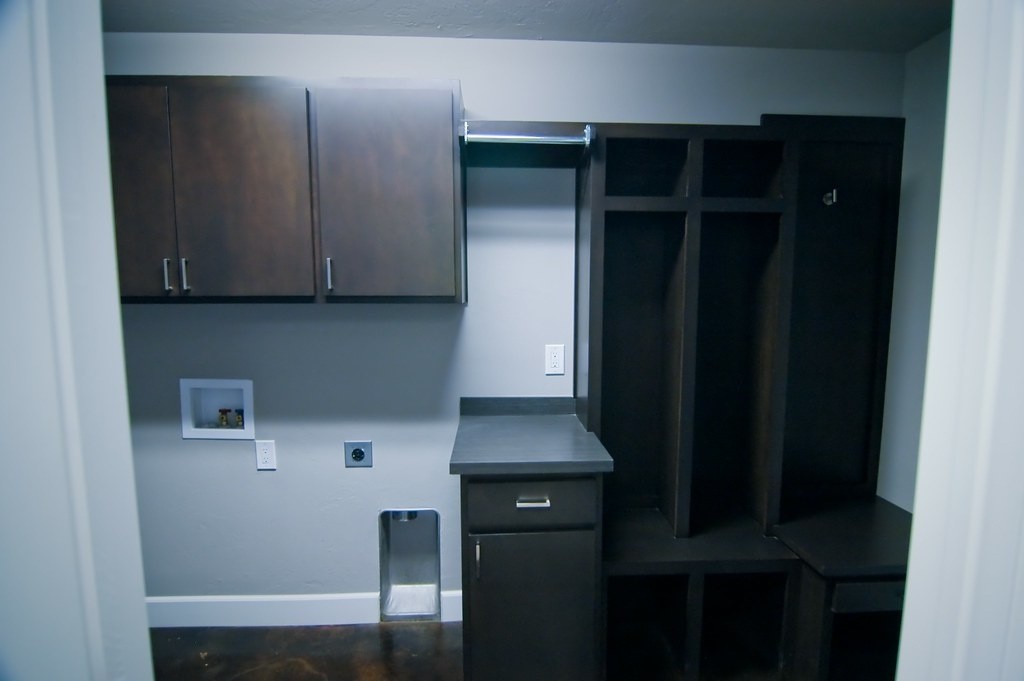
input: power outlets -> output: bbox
[545,345,565,375]
[255,439,276,470]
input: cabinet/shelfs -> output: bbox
[104,74,464,305]
[458,472,606,681]
[569,112,913,680]
